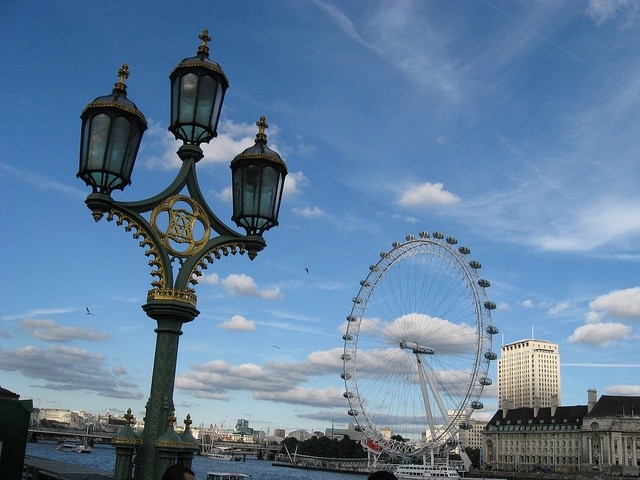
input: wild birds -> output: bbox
[272,345,280,349]
[85,306,95,316]
[304,267,309,274]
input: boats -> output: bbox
[207,451,233,462]
[54,442,93,454]
[206,471,251,480]
[393,463,462,480]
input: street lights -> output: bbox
[75,28,291,480]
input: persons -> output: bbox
[162,465,195,480]
